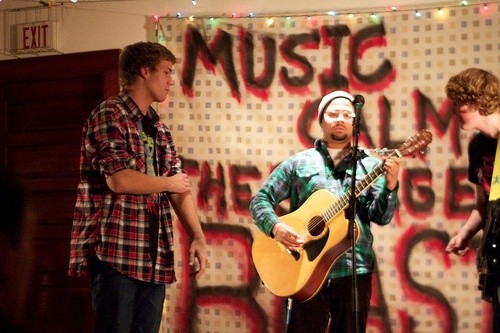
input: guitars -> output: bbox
[251,129,433,304]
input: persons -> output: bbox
[445,68,500,332]
[249,90,400,333]
[68,42,206,333]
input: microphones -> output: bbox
[352,94,365,136]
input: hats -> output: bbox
[317,91,355,126]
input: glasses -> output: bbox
[324,111,355,120]
